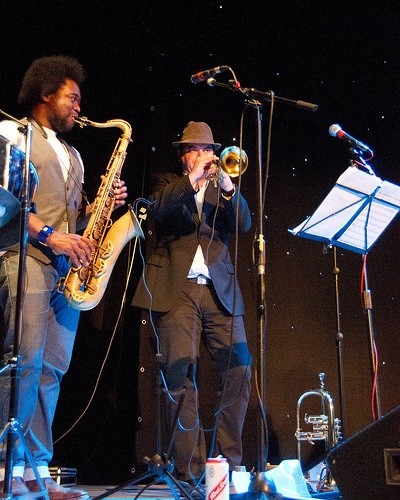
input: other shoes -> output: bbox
[26,477,90,499]
[1,476,37,499]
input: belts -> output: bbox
[187,274,211,284]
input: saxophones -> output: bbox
[56,116,145,311]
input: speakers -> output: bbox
[327,405,399,500]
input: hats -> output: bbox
[172,120,221,151]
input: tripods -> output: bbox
[95,355,203,500]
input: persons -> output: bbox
[131,121,252,500]
[0,55,128,500]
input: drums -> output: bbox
[0,133,40,250]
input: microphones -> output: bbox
[329,125,374,155]
[190,66,230,84]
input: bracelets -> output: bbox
[37,224,55,243]
[220,187,234,197]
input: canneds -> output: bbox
[205,454,229,500]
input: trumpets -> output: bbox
[295,372,342,493]
[206,146,249,187]
